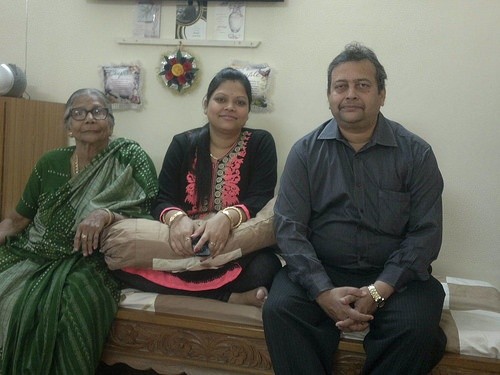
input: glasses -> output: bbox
[66,105,115,121]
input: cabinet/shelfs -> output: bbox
[0,95,69,223]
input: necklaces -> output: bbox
[211,153,219,161]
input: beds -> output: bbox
[101,275,500,375]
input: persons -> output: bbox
[109,67,283,307]
[262,42,448,375]
[0,89,159,375]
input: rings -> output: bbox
[81,233,87,238]
[211,242,216,246]
[184,237,190,241]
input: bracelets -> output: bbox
[218,209,234,228]
[103,207,111,227]
[225,205,242,227]
[168,211,187,227]
[112,211,115,222]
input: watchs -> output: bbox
[368,285,386,309]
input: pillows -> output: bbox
[100,196,277,273]
